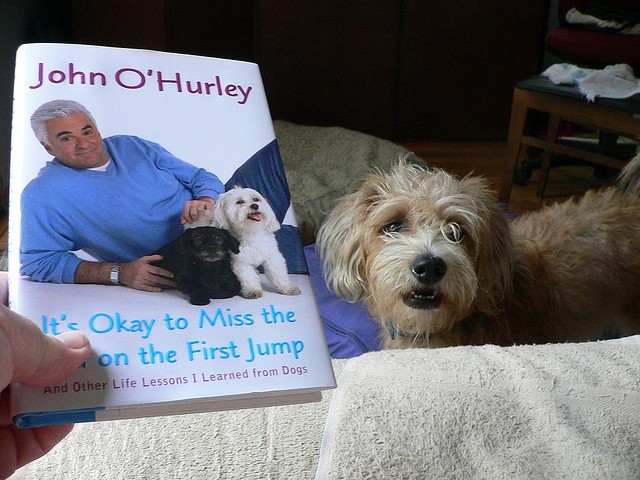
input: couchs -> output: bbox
[0,119,639,479]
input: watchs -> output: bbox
[110,262,123,285]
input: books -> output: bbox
[9,38,337,431]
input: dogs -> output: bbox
[138,226,241,306]
[182,185,302,300]
[314,150,640,350]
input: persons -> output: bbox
[0,271,93,480]
[20,99,309,293]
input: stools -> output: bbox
[497,74,639,213]
